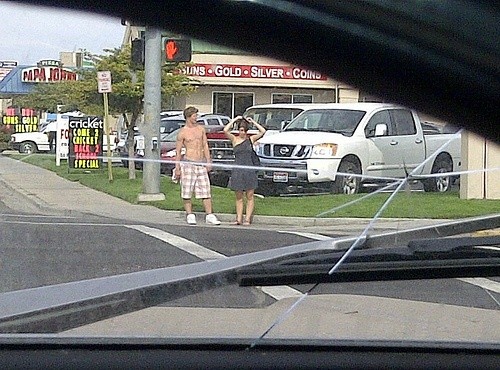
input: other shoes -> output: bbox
[186,213,197,225]
[230,220,242,225]
[205,213,221,225]
[243,221,251,226]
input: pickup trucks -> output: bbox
[8,121,117,154]
[254,102,461,195]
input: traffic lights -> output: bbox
[165,39,192,62]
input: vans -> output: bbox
[120,104,307,187]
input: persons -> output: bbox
[45,120,57,152]
[224,115,266,225]
[174,106,221,225]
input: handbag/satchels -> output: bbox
[252,150,260,167]
